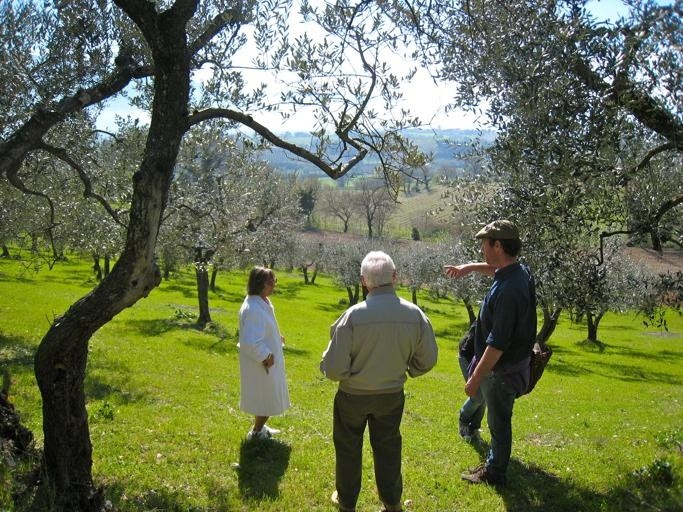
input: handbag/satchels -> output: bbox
[516,344,552,397]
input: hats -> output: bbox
[475,220,519,240]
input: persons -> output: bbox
[319,250,438,512]
[236,266,289,442]
[443,220,536,485]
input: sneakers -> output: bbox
[332,490,355,512]
[472,430,483,444]
[252,424,280,434]
[462,465,506,486]
[247,432,275,441]
[459,420,472,441]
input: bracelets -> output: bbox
[263,351,272,364]
